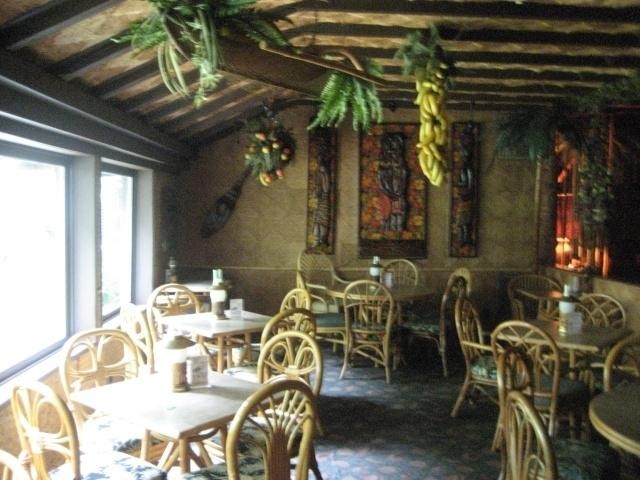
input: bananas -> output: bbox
[414,62,452,187]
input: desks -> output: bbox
[70,371,294,474]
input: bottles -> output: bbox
[557,283,575,336]
[210,269,227,321]
[166,334,197,392]
[168,255,178,282]
[369,255,383,283]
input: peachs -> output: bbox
[244,133,292,186]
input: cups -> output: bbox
[567,310,582,337]
[382,271,393,289]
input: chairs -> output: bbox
[117,283,324,437]
[451,271,640,480]
[1,377,168,480]
[177,374,316,480]
[295,246,472,385]
[205,330,322,478]
[58,327,168,461]
